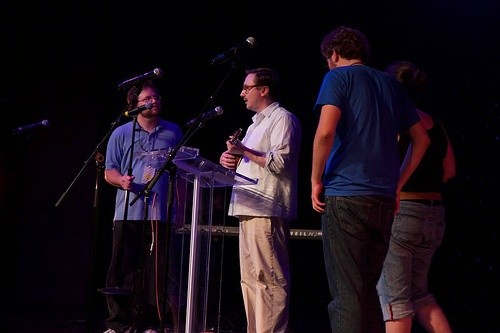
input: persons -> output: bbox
[309,27,429,333]
[374,60,455,333]
[220,67,303,333]
[104,81,187,333]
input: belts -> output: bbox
[412,199,443,207]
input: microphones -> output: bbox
[212,37,257,65]
[19,119,49,131]
[124,102,153,115]
[118,68,161,87]
[188,107,223,125]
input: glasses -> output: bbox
[138,96,163,102]
[240,84,266,93]
